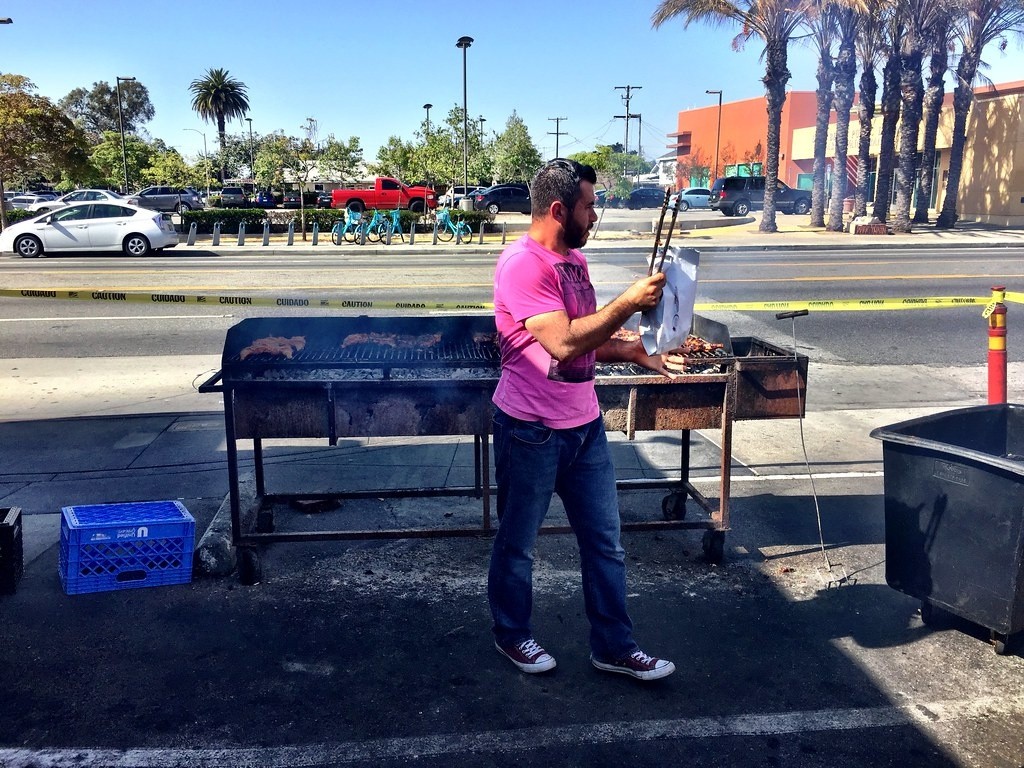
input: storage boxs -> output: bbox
[58,500,197,595]
[0,506,24,595]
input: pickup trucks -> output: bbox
[331,177,440,215]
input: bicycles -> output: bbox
[332,205,361,244]
[353,205,390,245]
[377,204,405,244]
[433,206,474,244]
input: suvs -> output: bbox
[708,175,815,216]
[122,186,206,217]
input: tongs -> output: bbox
[648,186,684,274]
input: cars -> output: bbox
[620,188,667,211]
[2,190,135,217]
[438,184,533,216]
[282,193,301,209]
[664,187,712,212]
[219,187,251,210]
[0,199,181,259]
[255,192,277,208]
[592,190,627,210]
[316,192,333,209]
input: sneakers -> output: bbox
[495,636,557,673]
[591,650,676,680]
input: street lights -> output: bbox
[117,77,139,195]
[628,113,643,191]
[480,118,487,150]
[423,103,432,135]
[183,127,209,202]
[244,116,254,185]
[704,89,724,181]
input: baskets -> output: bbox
[353,212,361,220]
[391,212,400,218]
[378,213,386,221]
[435,212,444,219]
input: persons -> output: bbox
[487,158,690,679]
